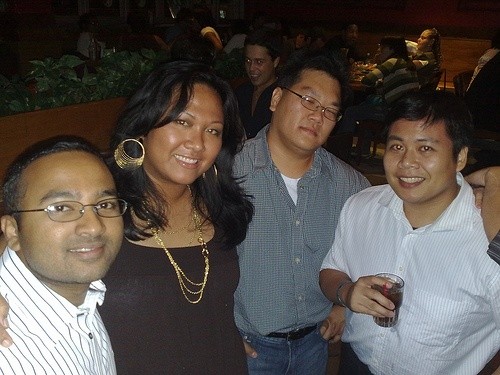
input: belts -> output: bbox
[267,323,318,341]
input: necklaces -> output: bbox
[141,184,211,303]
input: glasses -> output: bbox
[10,197,129,224]
[279,82,344,125]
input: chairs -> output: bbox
[355,69,443,160]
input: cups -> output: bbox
[370,273,405,328]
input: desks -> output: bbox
[349,81,384,95]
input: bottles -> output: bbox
[89,37,101,63]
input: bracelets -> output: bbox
[336,280,352,307]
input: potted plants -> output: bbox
[0,48,244,184]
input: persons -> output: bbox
[319,88,499,374]
[76,0,443,161]
[231,50,373,374]
[244,29,283,139]
[1,57,256,375]
[1,132,117,374]
